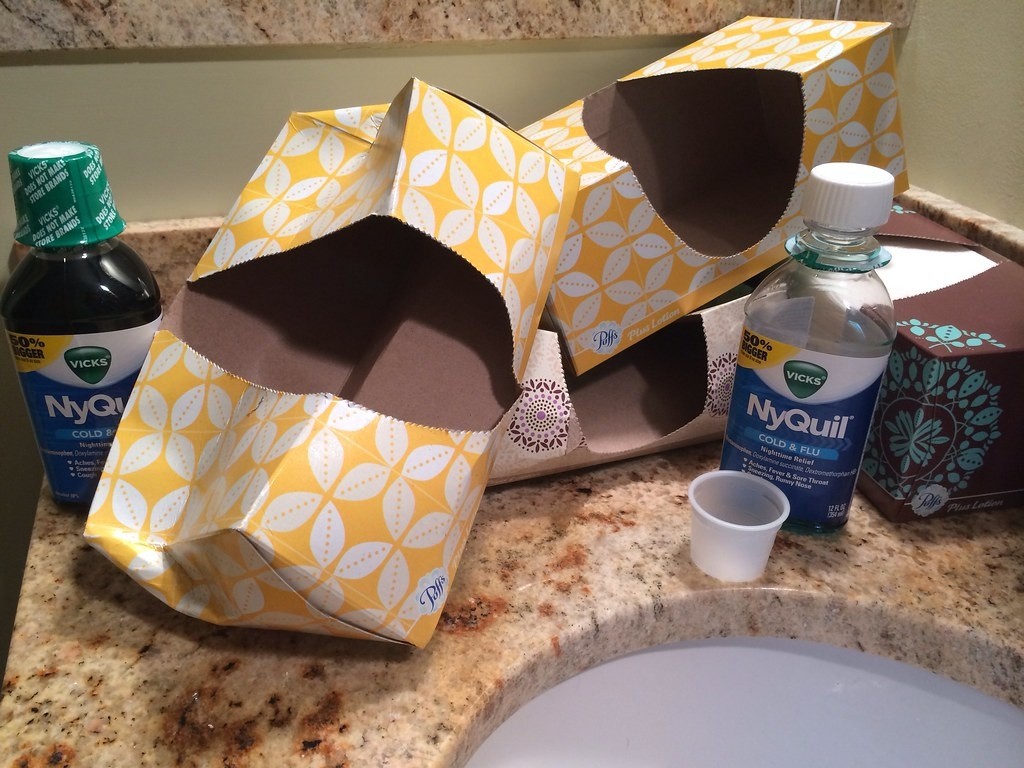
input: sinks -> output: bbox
[435,581,1023,768]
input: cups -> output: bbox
[684,471,791,580]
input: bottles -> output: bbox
[717,160,898,532]
[0,143,163,511]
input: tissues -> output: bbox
[804,200,1023,526]
